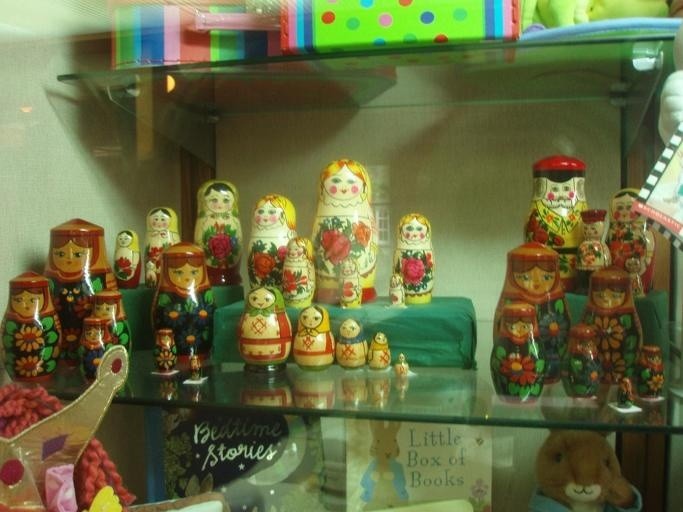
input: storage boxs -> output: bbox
[109,1,396,109]
[263,1,518,71]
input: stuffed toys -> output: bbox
[523,393,642,512]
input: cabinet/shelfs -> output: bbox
[42,37,682,511]
